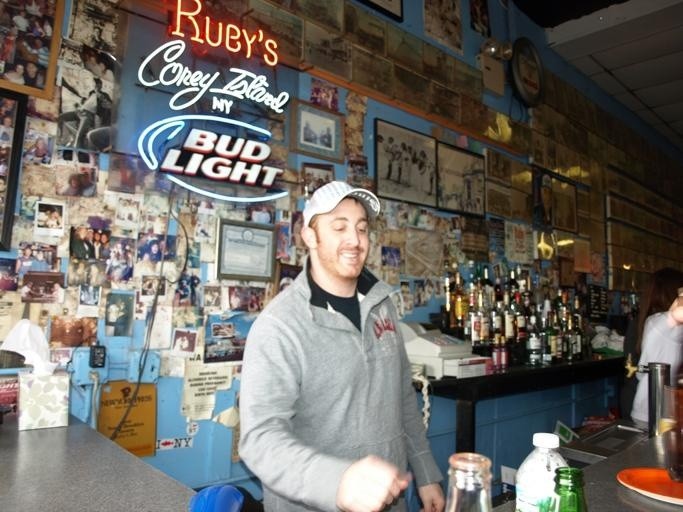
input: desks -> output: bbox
[415,320,630,463]
[0,414,198,512]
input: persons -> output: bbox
[1,208,239,347]
[630,268,683,425]
[0,10,114,198]
[239,179,445,511]
[299,113,335,150]
[377,118,438,203]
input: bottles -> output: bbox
[551,465,590,512]
[512,430,570,511]
[439,261,590,371]
[441,448,494,511]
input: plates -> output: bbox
[617,468,683,507]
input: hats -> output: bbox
[302,181,380,227]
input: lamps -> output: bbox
[478,37,514,60]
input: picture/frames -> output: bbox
[549,176,579,235]
[214,215,277,284]
[289,97,347,163]
[300,159,334,196]
[1,0,66,103]
[373,118,437,212]
[436,141,487,218]
[355,0,403,23]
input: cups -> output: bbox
[618,296,641,320]
